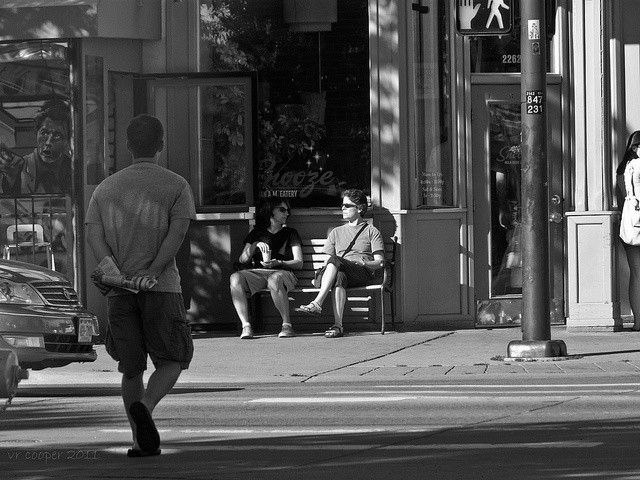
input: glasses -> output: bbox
[341,204,357,208]
[275,207,291,214]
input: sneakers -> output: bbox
[240,327,254,339]
[278,324,296,338]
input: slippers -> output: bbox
[128,400,161,451]
[127,442,162,457]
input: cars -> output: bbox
[0,258,97,370]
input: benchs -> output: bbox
[243,235,398,335]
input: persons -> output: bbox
[300,188,386,338]
[85,112,196,456]
[228,197,305,339]
[615,130,640,332]
[0,101,72,196]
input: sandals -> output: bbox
[300,302,322,317]
[325,324,343,338]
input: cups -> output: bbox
[261,250,273,263]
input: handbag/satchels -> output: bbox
[314,266,327,289]
[619,160,639,246]
